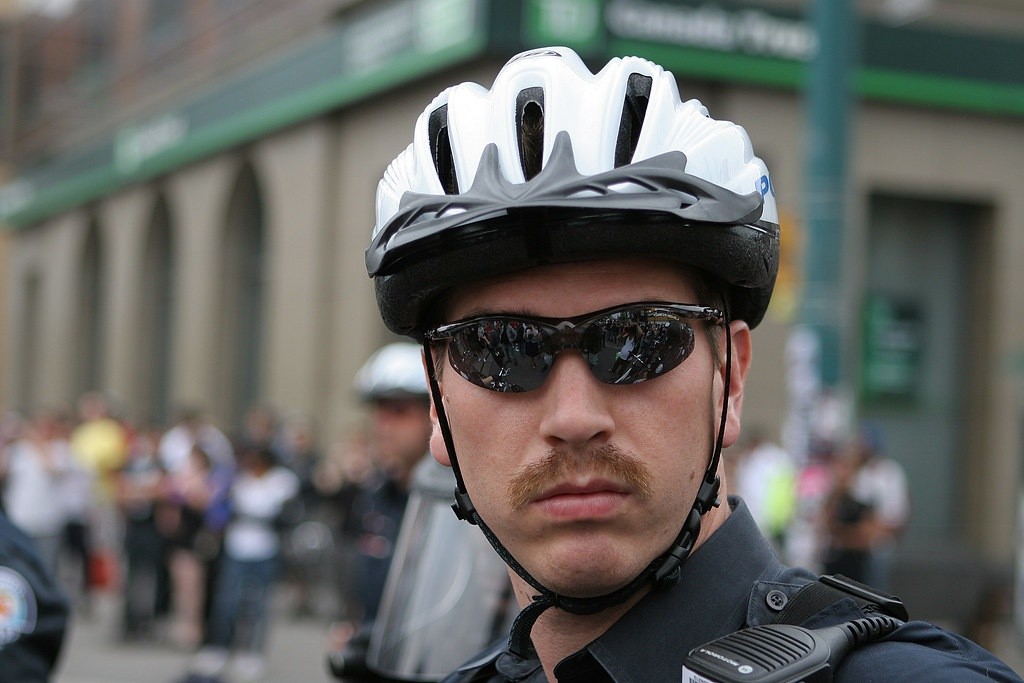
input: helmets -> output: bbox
[354,340,431,399]
[363,46,779,330]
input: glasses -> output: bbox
[413,300,728,393]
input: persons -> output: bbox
[0,340,907,683]
[364,46,1024,683]
